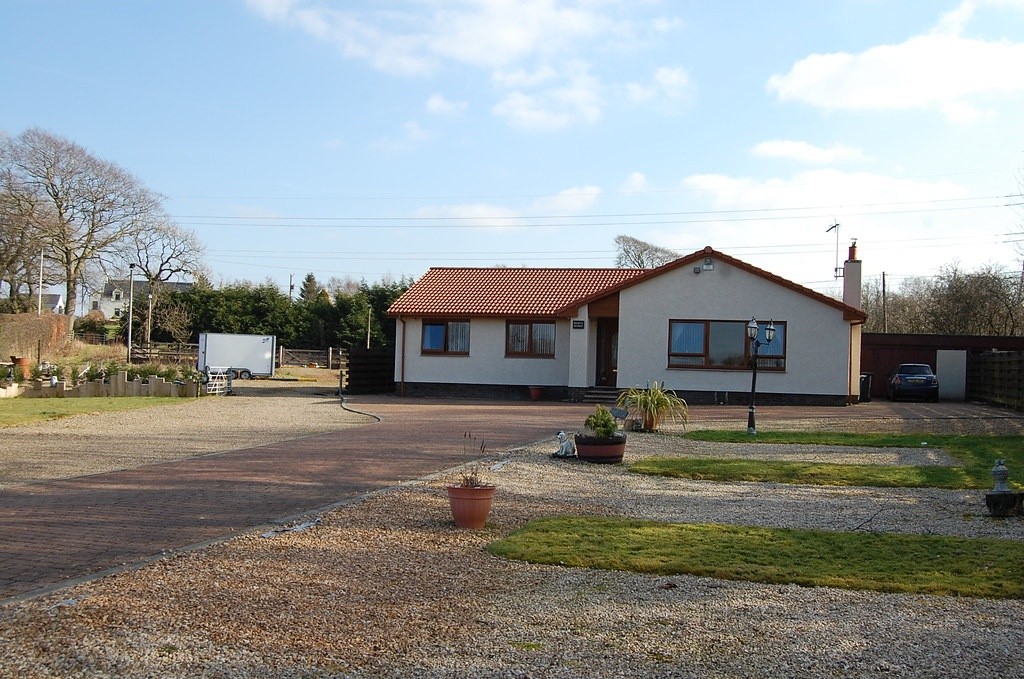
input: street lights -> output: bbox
[127,262,135,363]
[745,316,777,436]
[366,302,373,350]
[147,293,153,356]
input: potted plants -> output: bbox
[612,369,690,432]
[575,404,627,465]
[447,432,496,530]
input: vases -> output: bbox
[529,387,541,400]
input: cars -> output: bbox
[889,362,939,403]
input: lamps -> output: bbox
[746,317,776,346]
